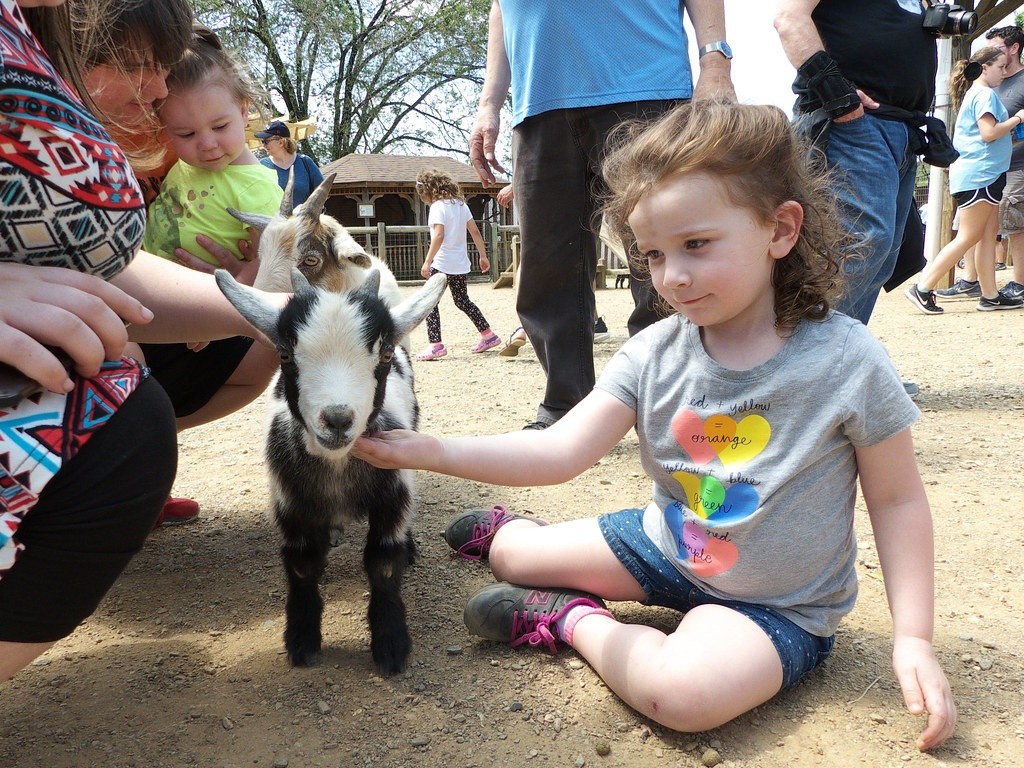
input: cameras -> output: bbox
[922,3,978,39]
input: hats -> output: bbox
[254,120,291,139]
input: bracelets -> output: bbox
[1013,115,1022,127]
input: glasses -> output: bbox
[261,139,272,144]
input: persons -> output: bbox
[254,120,325,213]
[0,0,284,680]
[496,183,610,357]
[415,169,501,360]
[350,98,958,753]
[773,0,938,399]
[903,25,1024,315]
[469,0,739,431]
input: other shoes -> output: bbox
[593,317,610,343]
[470,335,502,354]
[153,495,200,527]
[415,344,448,362]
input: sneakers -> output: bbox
[976,291,1024,312]
[443,504,551,561]
[996,281,1024,298]
[463,582,609,656]
[934,279,983,299]
[996,263,1007,271]
[904,283,945,315]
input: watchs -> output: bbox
[699,40,733,60]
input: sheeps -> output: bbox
[214,267,448,678]
[225,164,411,357]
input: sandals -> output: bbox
[498,326,529,358]
